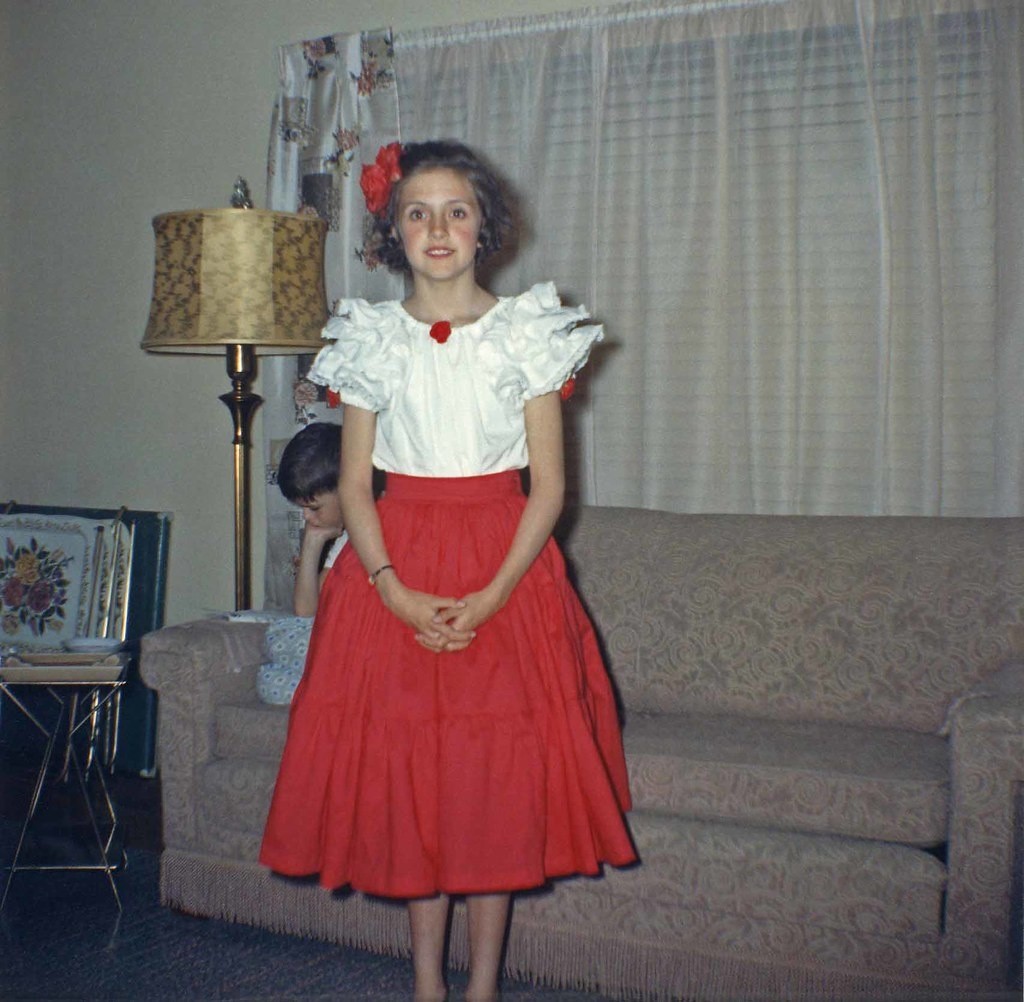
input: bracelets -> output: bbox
[367,564,393,584]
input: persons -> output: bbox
[276,421,350,620]
[259,137,642,1001]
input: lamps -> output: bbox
[139,175,339,624]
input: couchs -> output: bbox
[139,491,1024,999]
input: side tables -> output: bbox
[1,643,138,924]
